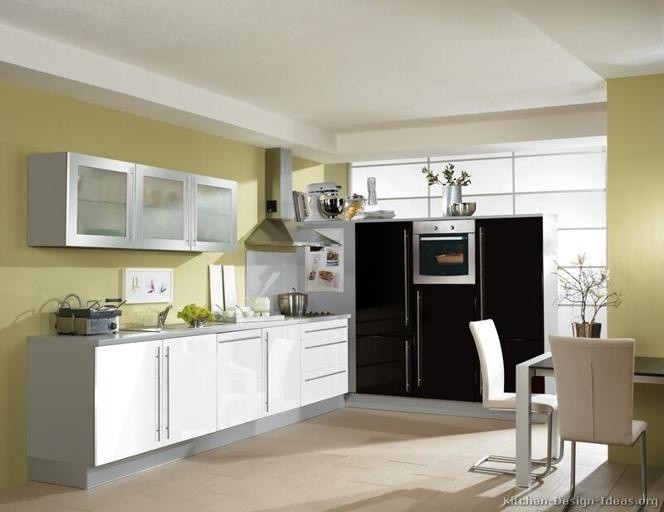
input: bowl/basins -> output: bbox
[221,311,235,317]
[242,312,253,317]
[452,203,477,216]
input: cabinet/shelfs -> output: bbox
[190,172,239,253]
[25,325,217,490]
[302,313,351,423]
[263,320,301,436]
[27,150,136,250]
[137,164,191,252]
[216,323,262,449]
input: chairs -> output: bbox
[467,318,564,479]
[545,335,647,512]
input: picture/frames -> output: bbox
[122,267,174,305]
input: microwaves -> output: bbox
[278,288,308,318]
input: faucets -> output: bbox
[158,304,173,328]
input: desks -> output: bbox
[515,352,663,489]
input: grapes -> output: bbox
[177,304,216,323]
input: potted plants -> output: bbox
[552,251,623,339]
[420,162,472,217]
